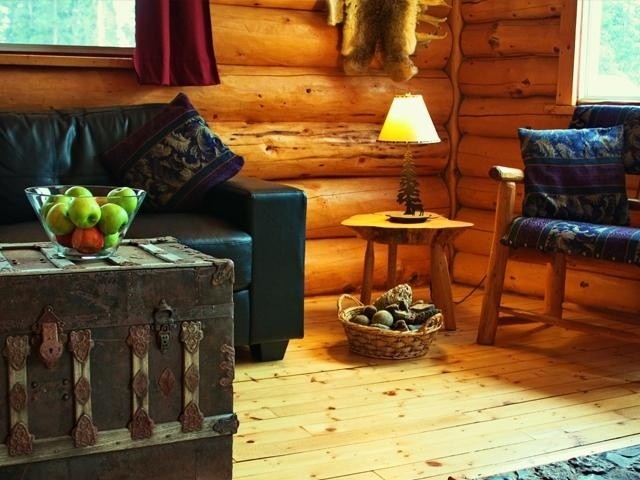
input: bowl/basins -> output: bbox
[25,183,147,261]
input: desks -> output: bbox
[342,209,474,334]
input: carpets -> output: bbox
[468,442,639,480]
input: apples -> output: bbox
[41,185,137,255]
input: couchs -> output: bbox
[3,103,307,364]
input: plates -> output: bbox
[386,213,432,222]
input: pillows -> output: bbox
[95,91,244,216]
[518,124,628,228]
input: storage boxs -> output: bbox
[1,234,236,479]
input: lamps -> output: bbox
[376,93,441,224]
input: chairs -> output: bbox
[478,103,640,346]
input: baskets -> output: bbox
[337,293,445,360]
